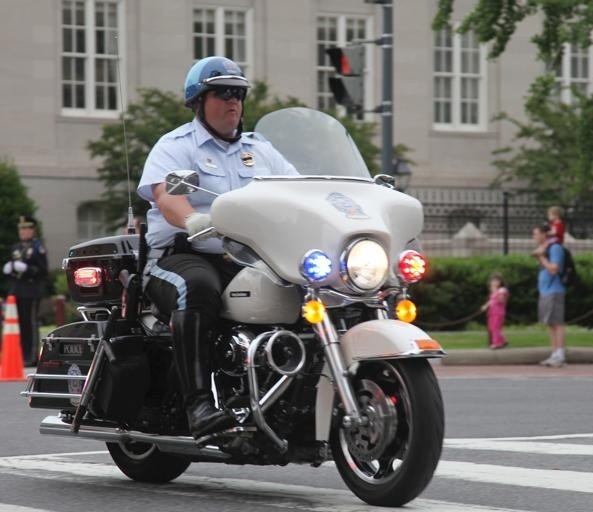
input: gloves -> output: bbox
[2,260,14,276]
[14,259,28,274]
[184,212,218,242]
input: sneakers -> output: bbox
[539,354,564,368]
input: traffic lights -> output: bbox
[320,39,367,116]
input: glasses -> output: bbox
[207,86,247,102]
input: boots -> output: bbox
[169,309,234,440]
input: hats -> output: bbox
[15,215,38,230]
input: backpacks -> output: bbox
[545,243,575,288]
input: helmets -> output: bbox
[183,56,252,108]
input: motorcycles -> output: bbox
[19,100,452,510]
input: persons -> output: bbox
[479,271,512,350]
[528,224,568,368]
[0,214,48,369]
[531,206,565,255]
[135,56,302,440]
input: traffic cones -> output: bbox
[0,294,34,385]
